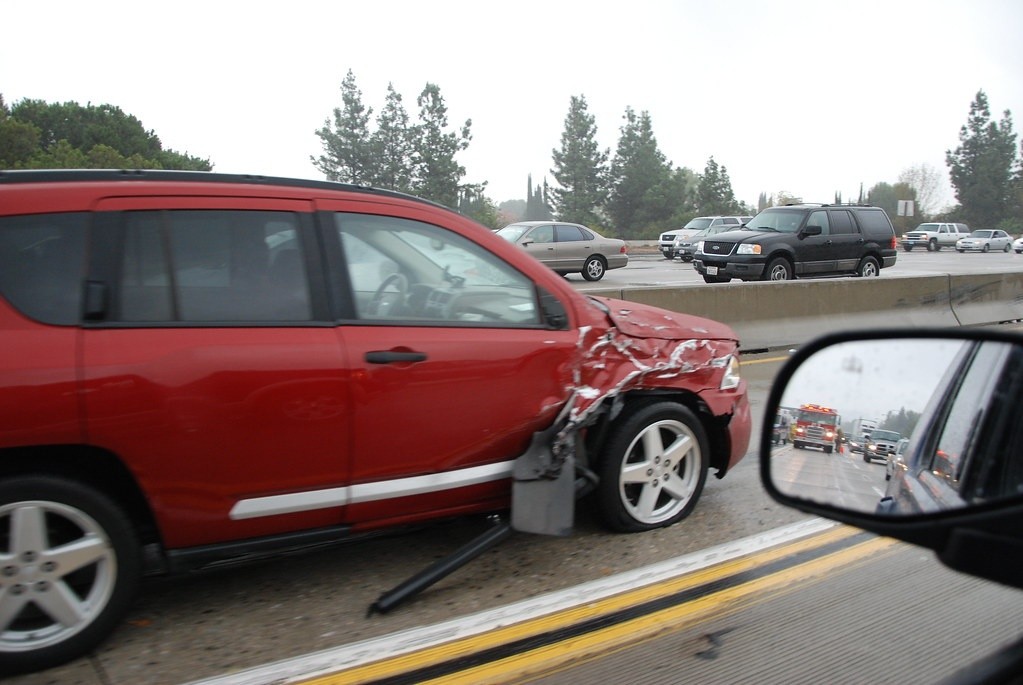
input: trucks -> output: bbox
[771,409,792,445]
[850,417,878,440]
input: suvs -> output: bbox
[863,430,900,463]
[692,202,898,284]
[657,216,754,259]
[901,223,971,252]
[0,169,752,681]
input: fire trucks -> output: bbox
[792,403,841,453]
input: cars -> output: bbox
[261,225,467,310]
[840,432,868,453]
[885,438,910,479]
[955,229,1014,253]
[675,223,741,262]
[1013,237,1023,253]
[490,221,629,282]
